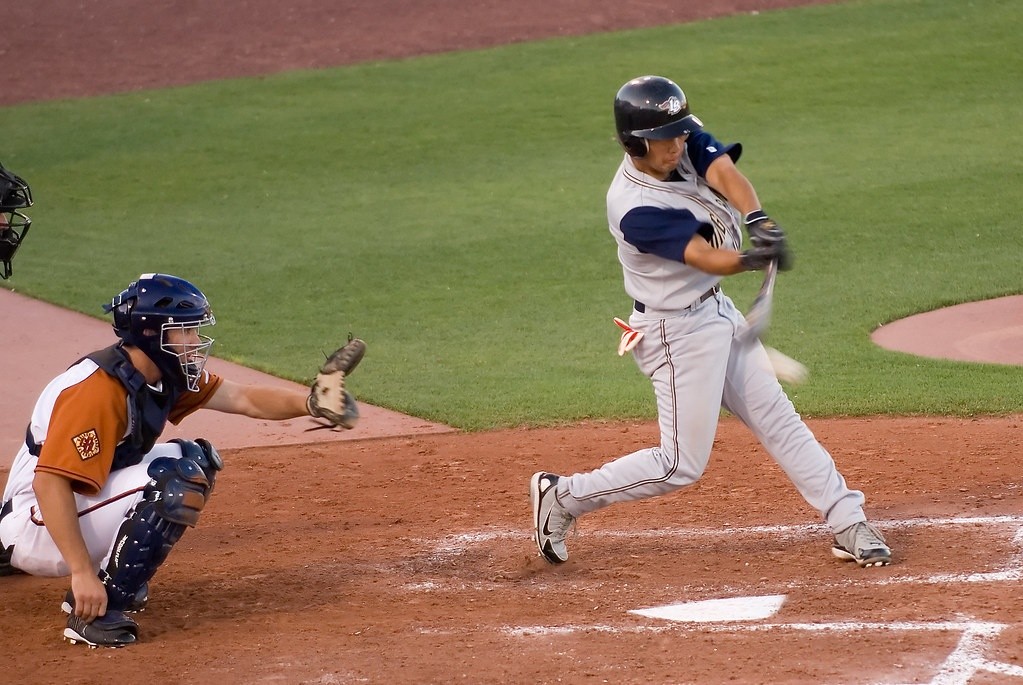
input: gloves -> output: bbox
[744,209,788,247]
[738,246,787,270]
[613,316,644,356]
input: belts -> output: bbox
[635,282,722,313]
[0,499,12,522]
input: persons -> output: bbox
[0,274,366,649]
[0,165,34,280]
[530,75,893,568]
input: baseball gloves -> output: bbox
[303,332,367,433]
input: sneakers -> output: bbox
[531,471,576,566]
[63,606,139,648]
[832,521,893,568]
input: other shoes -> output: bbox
[0,541,20,575]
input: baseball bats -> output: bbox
[738,256,779,349]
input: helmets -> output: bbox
[614,75,704,157]
[0,162,35,280]
[111,272,217,392]
